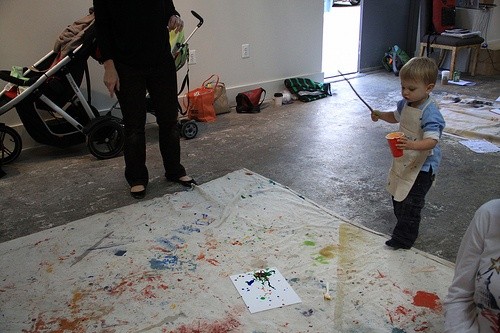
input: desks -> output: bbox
[420,42,481,80]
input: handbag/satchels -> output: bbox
[236,87,266,113]
[183,75,219,121]
[206,82,230,114]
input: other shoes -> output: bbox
[175,176,197,187]
[130,184,145,199]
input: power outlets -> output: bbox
[241,44,250,58]
[186,49,196,65]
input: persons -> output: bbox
[93,0,197,199]
[370,56,446,249]
[445,199,500,333]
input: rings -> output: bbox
[176,21,180,23]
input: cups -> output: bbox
[441,70,450,85]
[274,93,283,106]
[385,132,404,158]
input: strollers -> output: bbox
[0,7,125,166]
[103,10,204,140]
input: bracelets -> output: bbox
[175,15,180,18]
